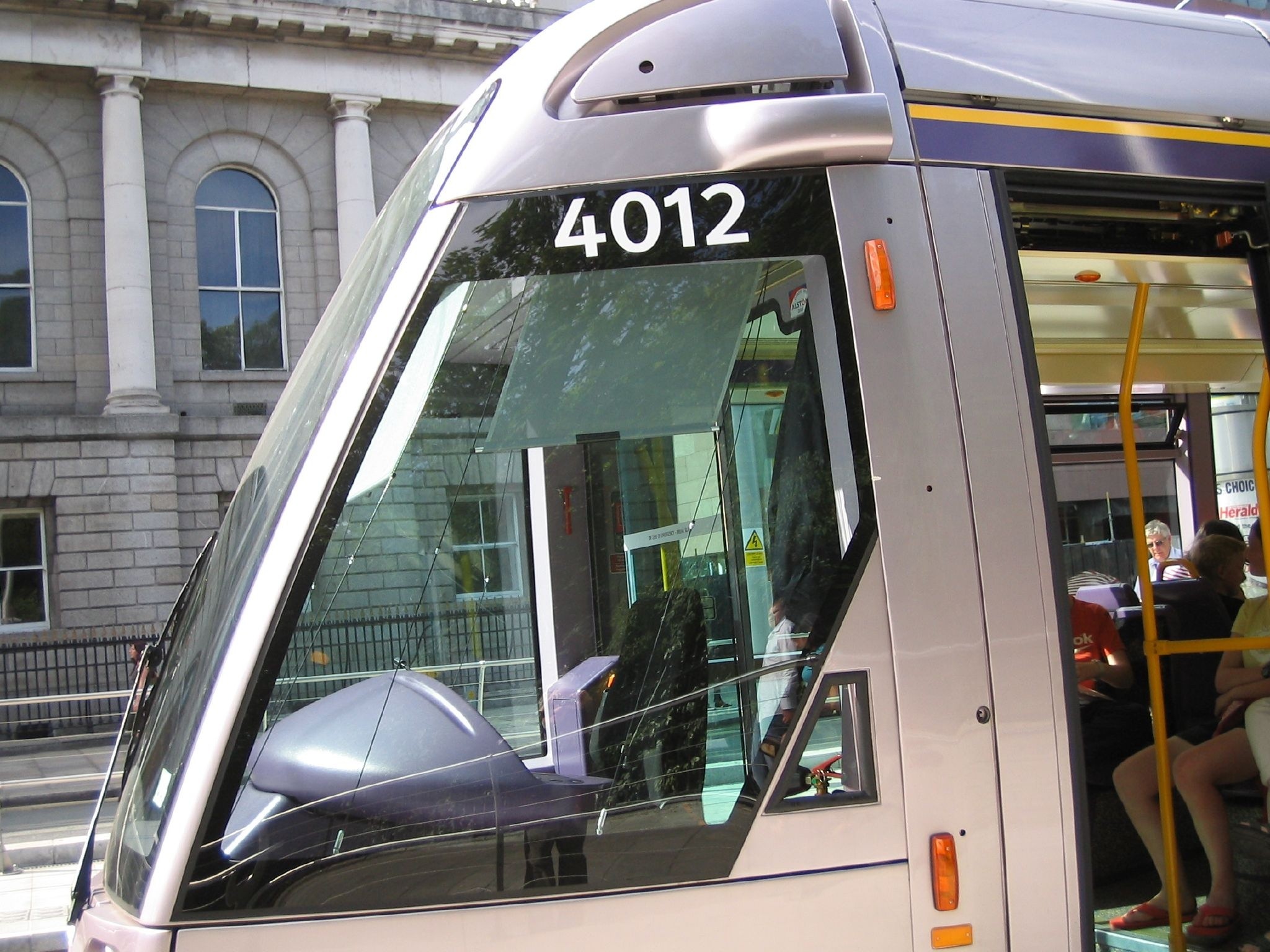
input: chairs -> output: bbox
[1076,558,1234,717]
[589,588,707,799]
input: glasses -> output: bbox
[1147,541,1164,548]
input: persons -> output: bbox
[698,586,735,711]
[751,598,825,791]
[1066,519,1270,949]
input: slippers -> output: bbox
[1187,903,1246,937]
[1112,903,1198,929]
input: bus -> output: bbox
[63,0,1270,952]
